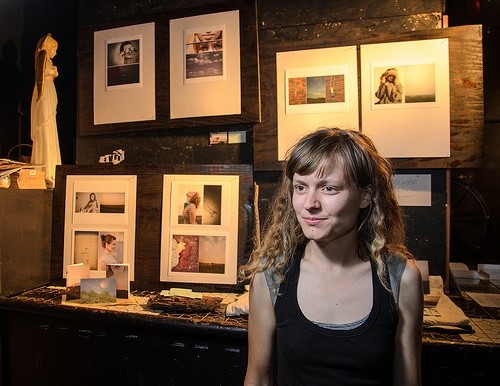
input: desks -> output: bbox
[3,283,500,385]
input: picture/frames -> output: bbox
[77,0,261,139]
[239,10,499,182]
[49,163,256,293]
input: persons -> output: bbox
[79,194,100,212]
[179,189,201,225]
[373,67,403,103]
[188,28,223,56]
[117,42,135,66]
[28,33,63,190]
[244,126,426,386]
[97,235,119,274]
